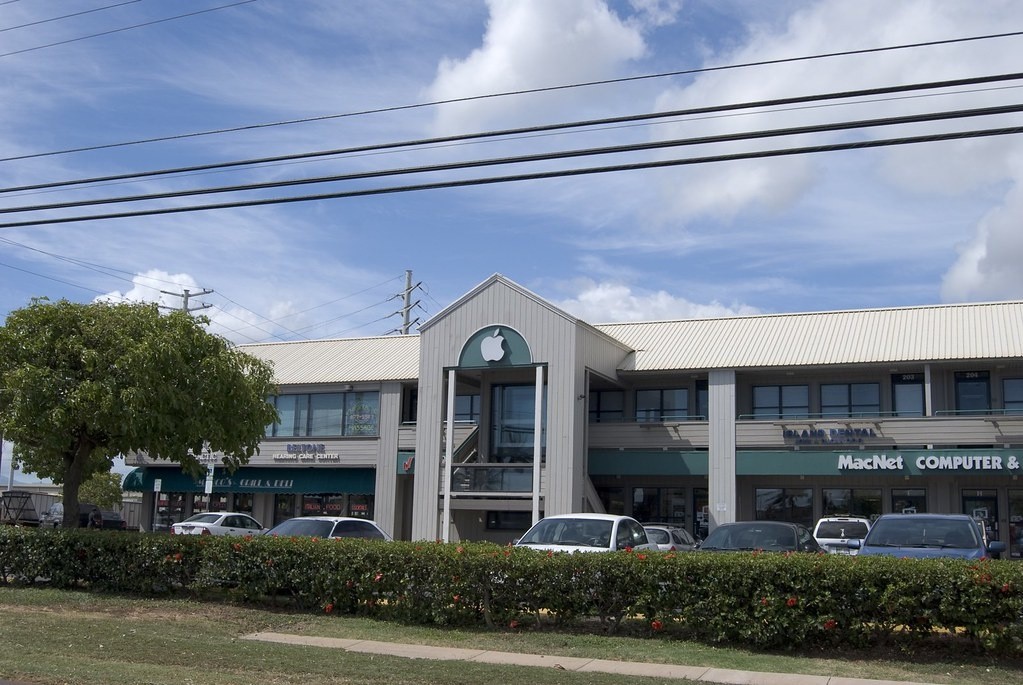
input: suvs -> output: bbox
[634,523,697,551]
[813,515,873,556]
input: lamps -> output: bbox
[578,394,586,400]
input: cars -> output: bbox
[39,502,103,530]
[170,512,271,537]
[694,521,827,554]
[262,516,395,543]
[101,512,127,531]
[847,513,1008,560]
[513,513,660,611]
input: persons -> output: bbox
[87,508,102,529]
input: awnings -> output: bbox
[122,466,376,496]
